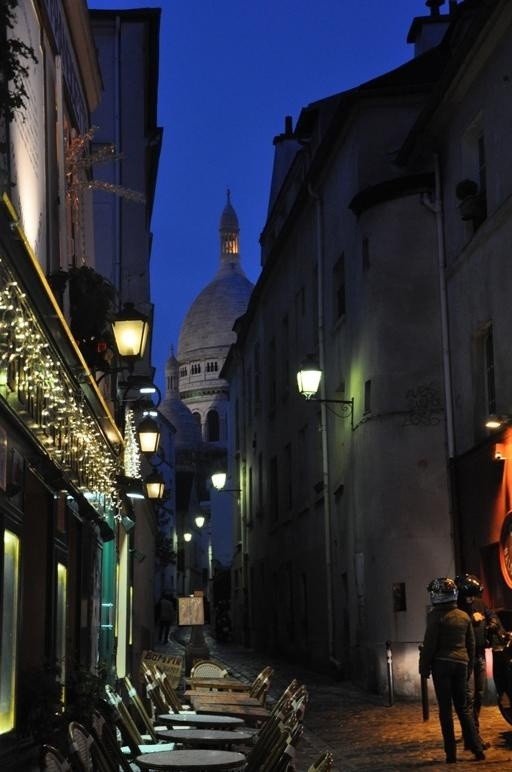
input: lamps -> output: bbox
[296,354,354,432]
[209,467,242,496]
[145,468,166,503]
[136,413,164,457]
[129,547,146,566]
[117,515,136,535]
[96,302,151,383]
[484,410,512,430]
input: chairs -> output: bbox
[39,660,336,772]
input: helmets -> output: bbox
[427,577,458,604]
[454,574,483,604]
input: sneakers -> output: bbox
[464,738,490,759]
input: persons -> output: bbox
[154,592,175,643]
[418,575,485,763]
[455,573,508,751]
